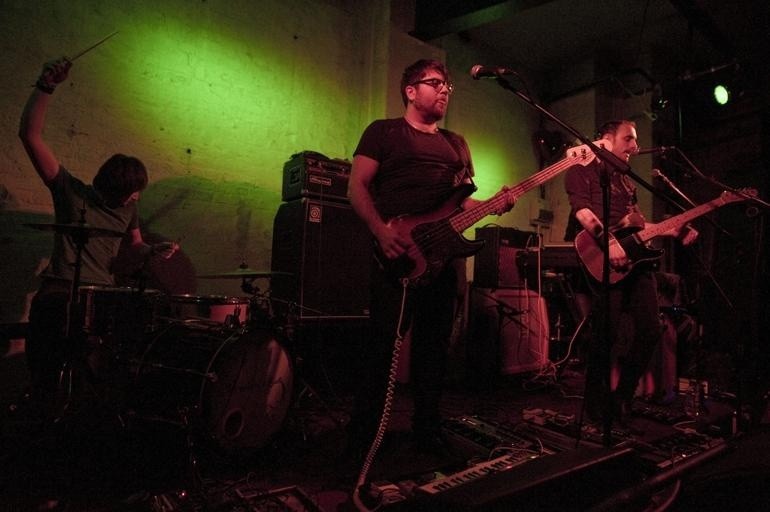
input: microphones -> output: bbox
[467,59,509,81]
[629,143,667,159]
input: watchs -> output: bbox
[36,79,56,95]
[592,226,604,240]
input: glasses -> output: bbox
[409,78,455,93]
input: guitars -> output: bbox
[571,185,758,283]
[376,138,616,283]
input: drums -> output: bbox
[166,293,252,331]
[115,314,296,466]
[76,284,162,340]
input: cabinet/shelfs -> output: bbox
[476,228,544,292]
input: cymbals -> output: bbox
[192,267,297,281]
[21,220,133,241]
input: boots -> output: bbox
[580,373,611,443]
[614,360,645,434]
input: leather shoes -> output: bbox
[418,435,467,465]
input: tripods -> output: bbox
[668,146,770,442]
[574,164,646,451]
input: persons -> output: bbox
[348,59,518,479]
[564,119,680,432]
[18,59,179,415]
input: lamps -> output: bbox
[714,84,742,105]
